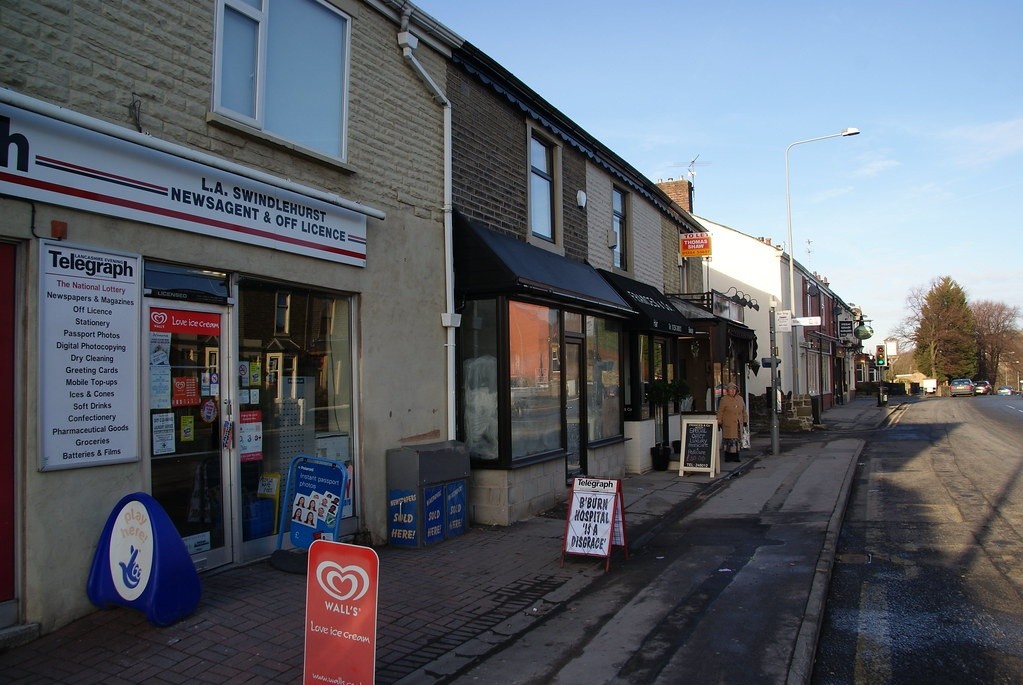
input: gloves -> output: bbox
[743,422,748,427]
[718,424,722,431]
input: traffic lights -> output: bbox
[876,345,888,366]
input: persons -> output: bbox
[716,381,750,463]
[292,491,339,526]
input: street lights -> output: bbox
[786,127,859,395]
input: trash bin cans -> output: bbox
[386,440,472,549]
[878,387,888,406]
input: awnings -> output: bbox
[457,212,696,334]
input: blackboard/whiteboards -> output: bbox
[680,419,719,472]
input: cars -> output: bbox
[949,379,975,397]
[997,386,1014,396]
[973,381,992,395]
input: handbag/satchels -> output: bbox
[741,427,751,449]
